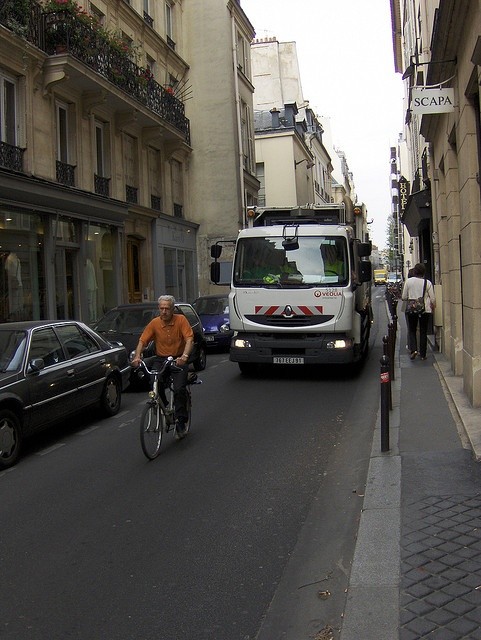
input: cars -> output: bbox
[0,318,131,468]
[89,301,207,387]
[192,293,233,353]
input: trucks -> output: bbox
[209,203,374,375]
[373,269,388,287]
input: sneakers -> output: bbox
[422,356,428,361]
[410,350,418,360]
[176,416,188,433]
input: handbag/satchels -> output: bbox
[406,297,425,315]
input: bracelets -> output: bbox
[183,354,189,358]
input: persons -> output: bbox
[319,246,357,280]
[401,269,421,354]
[131,295,195,424]
[401,262,437,360]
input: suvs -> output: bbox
[387,272,402,288]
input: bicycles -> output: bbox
[132,355,192,460]
